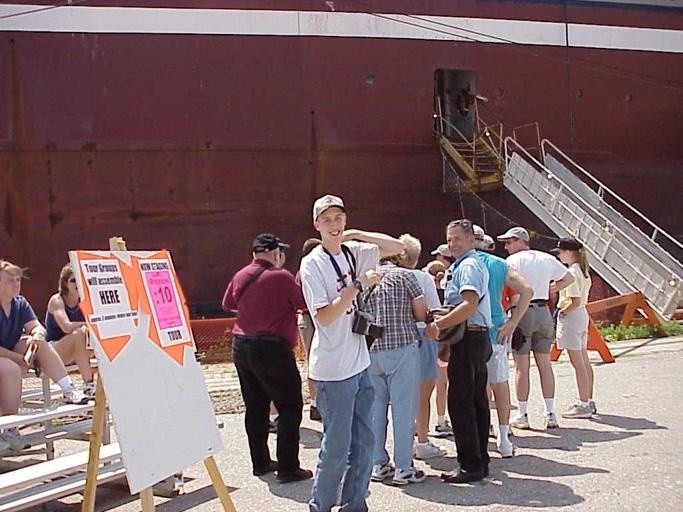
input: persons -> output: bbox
[45,263,96,395]
[0,260,88,449]
[219,189,598,512]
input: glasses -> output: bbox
[69,278,76,282]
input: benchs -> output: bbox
[0,359,224,512]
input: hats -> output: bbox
[496,226,530,242]
[431,244,452,257]
[312,194,345,222]
[481,234,495,251]
[302,238,322,256]
[421,266,436,279]
[473,224,485,248]
[252,232,288,252]
[549,238,580,252]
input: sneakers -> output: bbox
[508,424,513,436]
[543,412,560,428]
[509,413,530,429]
[496,443,515,458]
[412,440,447,460]
[253,460,278,476]
[561,401,597,419]
[370,463,395,482]
[310,405,321,420]
[61,383,89,404]
[435,420,455,438]
[392,461,427,485]
[269,415,280,432]
[277,468,313,480]
[488,423,494,437]
[0,427,31,450]
[84,382,95,396]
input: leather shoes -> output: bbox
[440,464,489,483]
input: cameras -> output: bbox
[352,310,386,336]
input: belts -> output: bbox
[529,304,546,307]
[236,335,288,345]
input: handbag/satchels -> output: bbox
[423,307,467,345]
[511,327,526,351]
